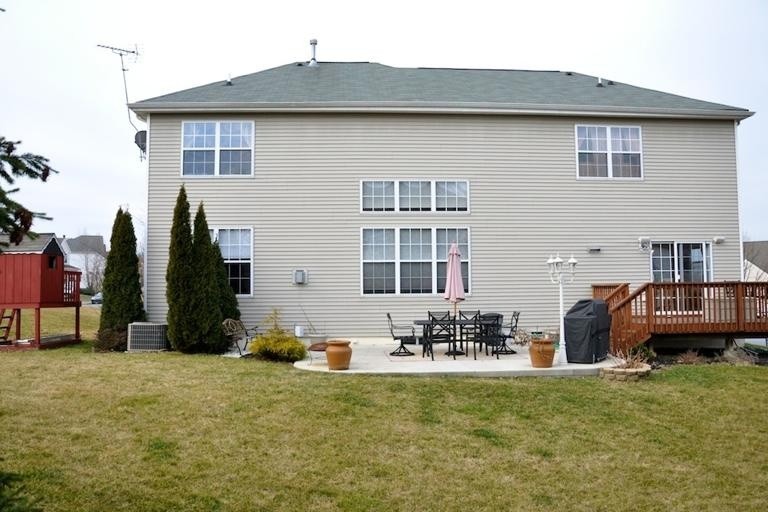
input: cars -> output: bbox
[91,292,103,304]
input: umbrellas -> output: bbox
[443,242,465,315]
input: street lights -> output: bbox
[545,249,578,365]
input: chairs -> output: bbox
[387,309,520,361]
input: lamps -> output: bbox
[638,237,650,251]
[714,236,725,243]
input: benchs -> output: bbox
[223,319,263,355]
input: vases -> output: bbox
[309,334,328,346]
[326,341,351,370]
[530,340,555,367]
